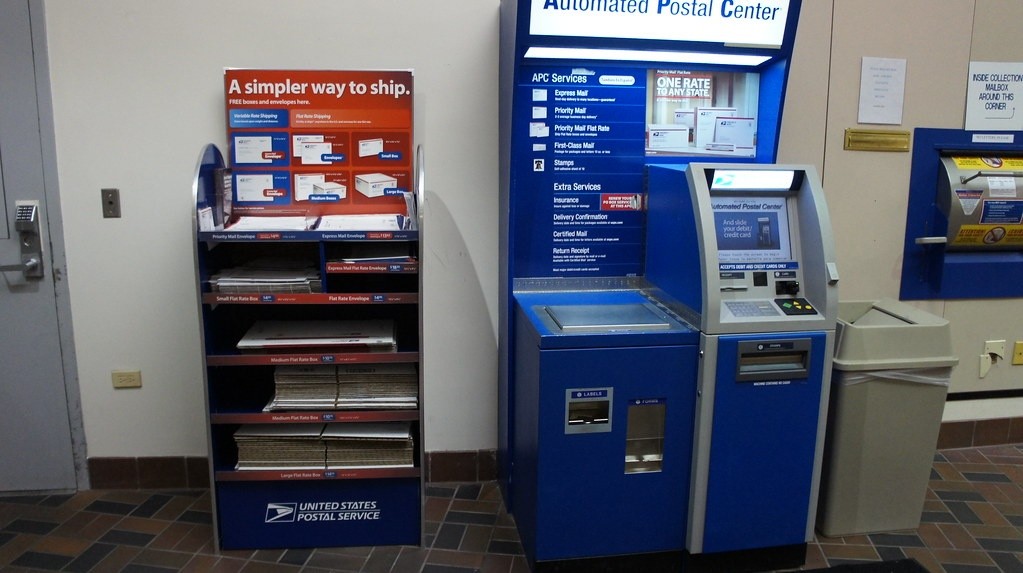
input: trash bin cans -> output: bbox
[817,300,962,539]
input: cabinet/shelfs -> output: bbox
[192,143,425,550]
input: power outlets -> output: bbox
[112,371,142,388]
